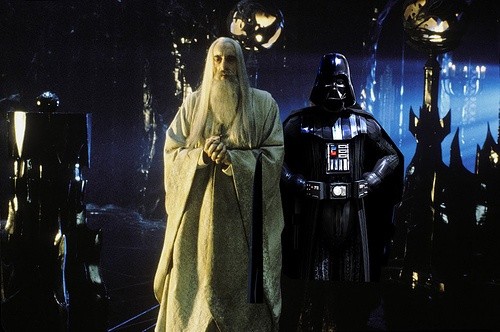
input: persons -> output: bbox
[280,51,405,332]
[153,36,287,332]
